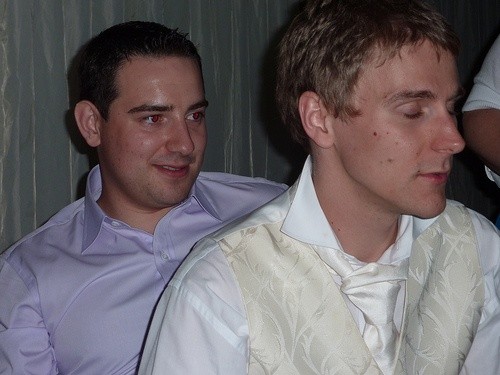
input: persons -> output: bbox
[0,20,291,375]
[461,33,500,230]
[134,0,500,374]
